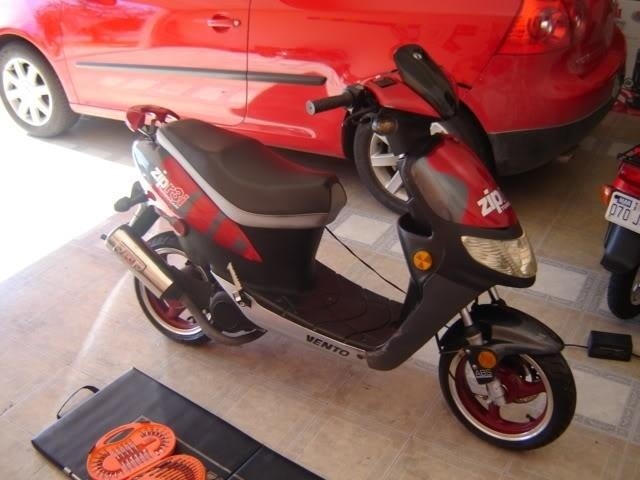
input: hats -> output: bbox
[0,0,627,216]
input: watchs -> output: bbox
[101,44,577,451]
[598,144,639,320]
